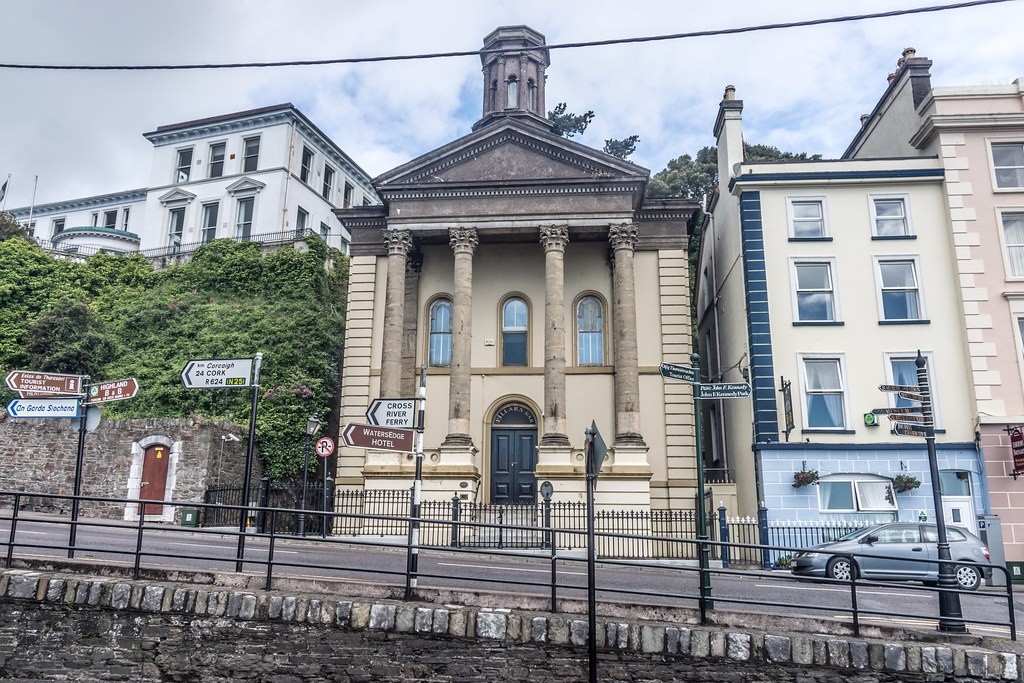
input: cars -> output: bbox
[790,521,993,592]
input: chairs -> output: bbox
[905,532,915,542]
[892,532,902,542]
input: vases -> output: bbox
[795,473,813,484]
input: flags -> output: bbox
[179,171,188,183]
[0,179,10,201]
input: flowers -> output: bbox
[792,470,820,488]
[893,475,920,494]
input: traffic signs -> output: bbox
[4,398,80,418]
[363,397,417,431]
[700,382,753,400]
[178,356,254,390]
[658,361,695,385]
[871,384,927,436]
[340,421,416,454]
[89,377,139,404]
[6,370,82,396]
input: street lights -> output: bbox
[293,411,323,536]
